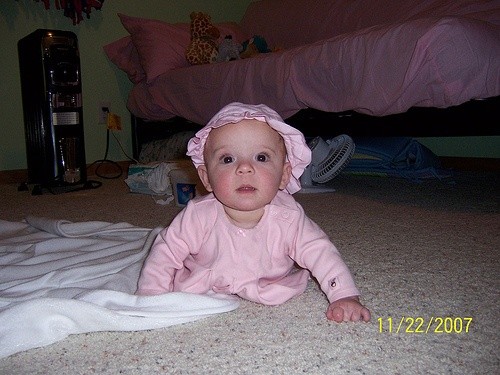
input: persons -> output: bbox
[135,101,372,324]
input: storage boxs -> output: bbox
[128,162,207,209]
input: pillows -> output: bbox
[103,13,247,86]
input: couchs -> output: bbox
[127,0,500,163]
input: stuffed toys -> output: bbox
[185,11,273,66]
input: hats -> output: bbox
[186,102,312,195]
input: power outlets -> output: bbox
[97,101,111,123]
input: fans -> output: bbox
[300,135,358,193]
